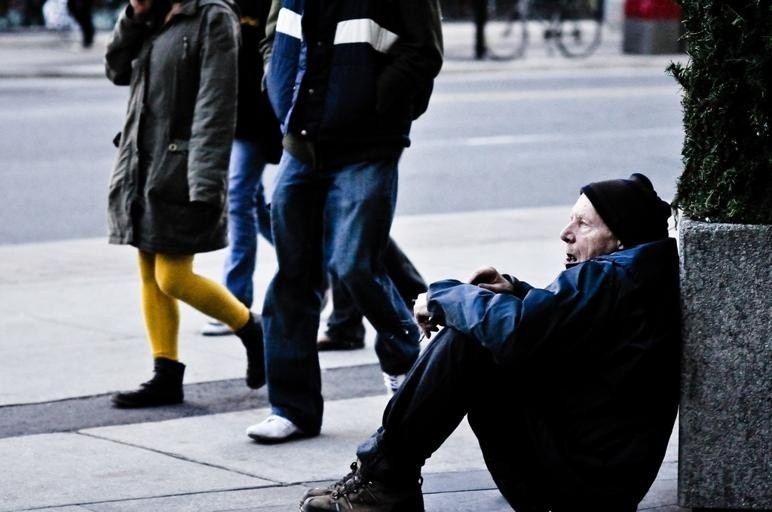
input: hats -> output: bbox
[581,173,672,247]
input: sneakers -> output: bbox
[301,478,425,512]
[201,318,232,334]
[318,337,365,350]
[383,375,405,396]
[246,413,321,443]
[300,466,359,502]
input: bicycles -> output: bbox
[483,1,605,61]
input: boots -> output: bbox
[110,358,185,409]
[233,311,264,391]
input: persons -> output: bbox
[0,0,127,61]
[102,1,272,409]
[198,0,293,337]
[294,171,687,511]
[246,0,441,445]
